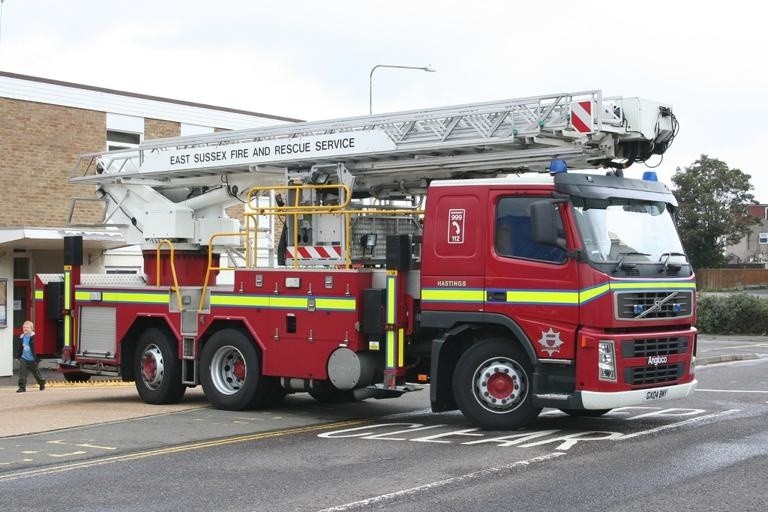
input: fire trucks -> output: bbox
[34,88,699,430]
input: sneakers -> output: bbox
[40,379,46,391]
[16,388,27,392]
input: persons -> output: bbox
[15,320,47,392]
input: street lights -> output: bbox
[369,65,438,114]
